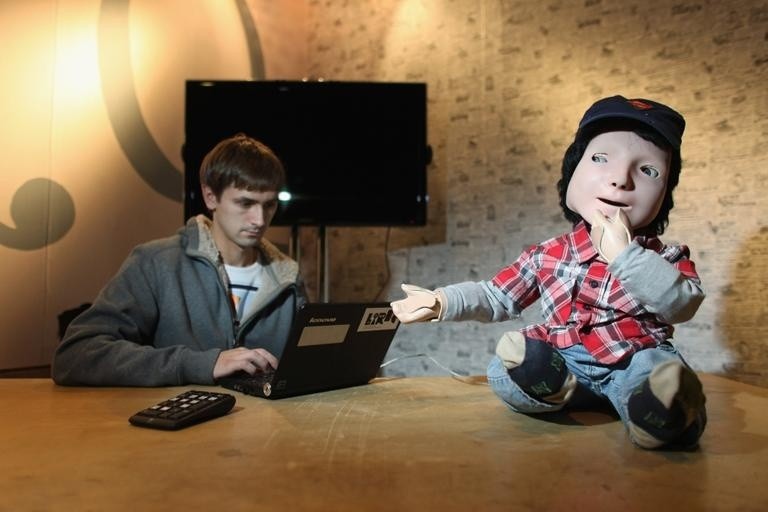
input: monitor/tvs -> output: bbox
[183,79,429,228]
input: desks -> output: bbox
[2,367,768,512]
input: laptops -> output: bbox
[218,302,402,400]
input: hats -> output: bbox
[577,95,685,144]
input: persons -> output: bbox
[385,90,714,450]
[45,136,310,389]
